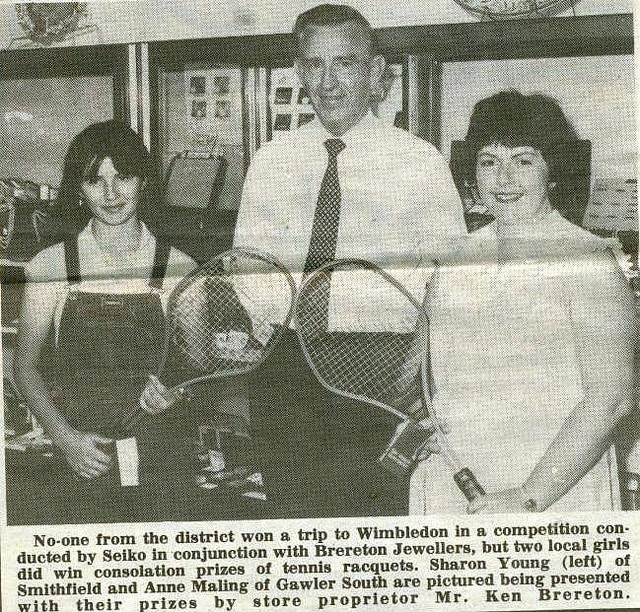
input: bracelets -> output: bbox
[519,487,537,513]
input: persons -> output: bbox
[13,120,207,523]
[230,3,469,518]
[407,87,640,516]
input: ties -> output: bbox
[300,139,346,346]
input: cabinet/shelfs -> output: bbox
[416,14,639,266]
[3,45,138,278]
[139,26,415,264]
